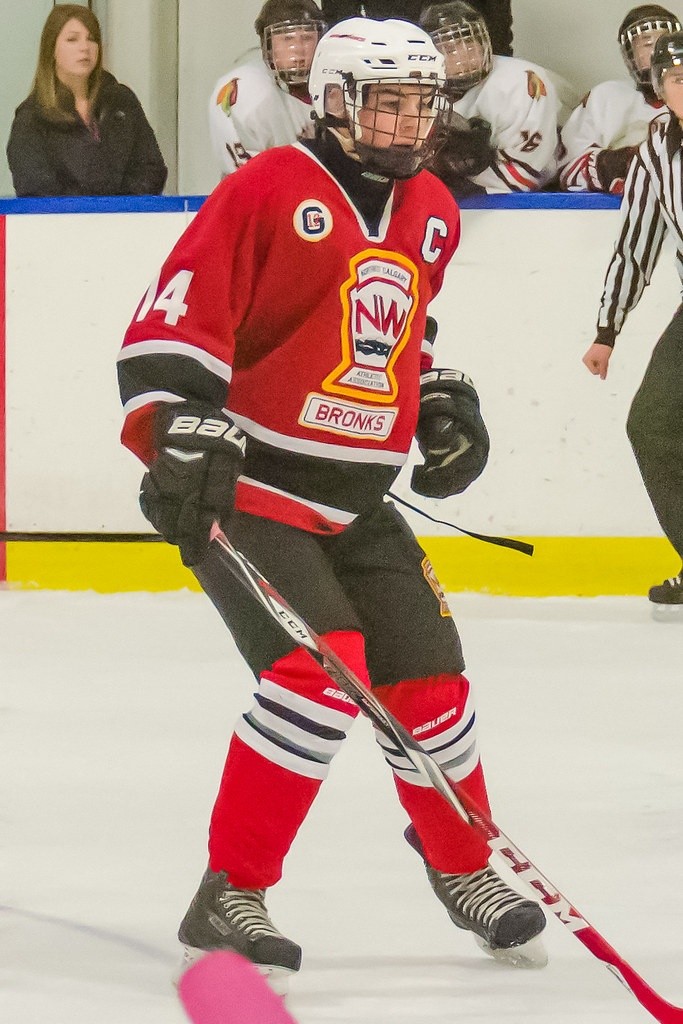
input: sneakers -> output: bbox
[404,822,549,967]
[174,868,302,994]
[649,569,683,622]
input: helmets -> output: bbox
[255,1,327,84]
[619,4,681,60]
[419,0,493,91]
[651,30,683,78]
[309,16,454,180]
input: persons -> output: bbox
[416,1,578,197]
[208,0,329,175]
[7,5,168,197]
[557,3,683,195]
[117,16,548,993]
[581,30,682,622]
[321,0,514,57]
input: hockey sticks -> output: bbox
[205,517,683,1024]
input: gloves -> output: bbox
[587,146,637,195]
[411,366,489,499]
[138,405,246,567]
